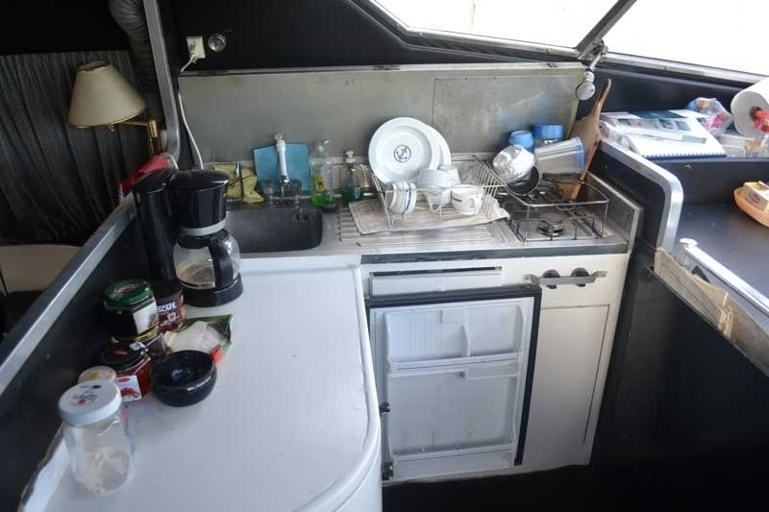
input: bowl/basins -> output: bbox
[492,145,536,185]
[150,348,216,407]
[508,166,542,197]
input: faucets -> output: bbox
[274,133,290,196]
[224,159,245,205]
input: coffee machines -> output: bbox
[133,168,183,306]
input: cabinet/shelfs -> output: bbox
[507,254,630,473]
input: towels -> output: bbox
[347,175,510,234]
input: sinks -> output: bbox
[225,202,338,259]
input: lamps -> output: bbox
[63,63,163,143]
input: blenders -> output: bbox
[169,170,243,308]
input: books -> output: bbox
[600,108,727,160]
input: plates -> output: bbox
[429,127,452,171]
[733,187,769,228]
[367,117,439,187]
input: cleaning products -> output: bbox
[308,140,336,211]
[339,149,364,208]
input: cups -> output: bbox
[385,182,416,215]
[451,183,484,216]
[534,135,585,174]
[418,168,447,190]
